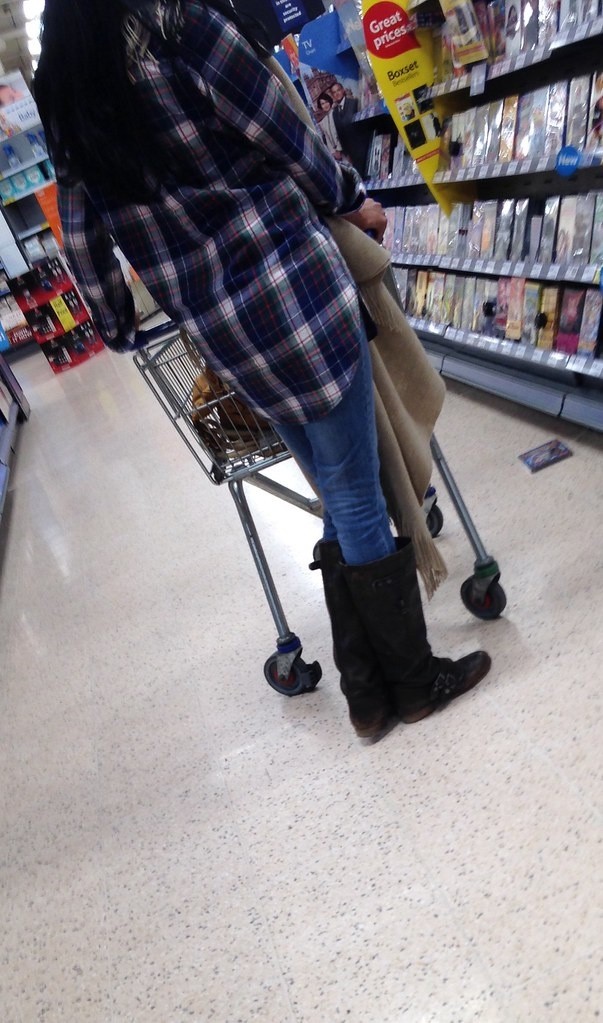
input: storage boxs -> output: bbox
[5,234,105,375]
[44,160,55,180]
[23,166,45,187]
[0,178,16,200]
[10,172,28,194]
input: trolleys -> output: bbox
[133,244,507,697]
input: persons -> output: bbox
[318,82,374,174]
[36,0,494,745]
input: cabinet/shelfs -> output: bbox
[345,1,603,432]
[1,70,93,318]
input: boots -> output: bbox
[320,538,402,745]
[337,538,491,723]
